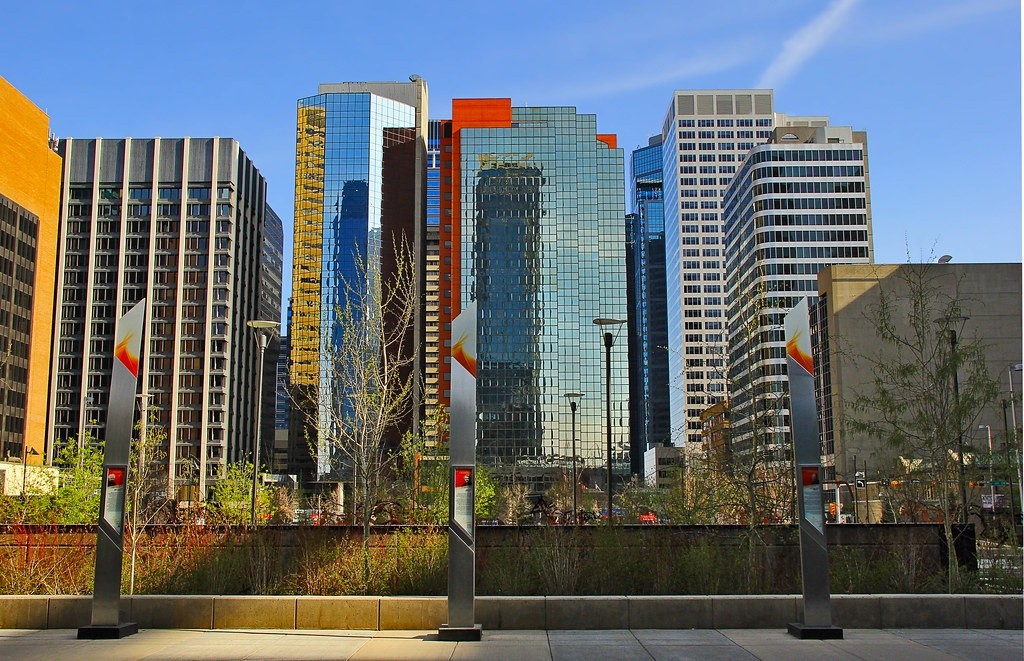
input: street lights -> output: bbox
[979,424,996,540]
[593,317,629,526]
[563,393,586,523]
[1007,364,1023,515]
[848,447,859,523]
[245,320,282,522]
[931,314,970,522]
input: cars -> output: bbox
[480,519,500,525]
[892,501,965,523]
[286,507,351,524]
[978,546,1022,593]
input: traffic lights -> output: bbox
[969,480,980,488]
[881,478,891,486]
[830,503,836,515]
